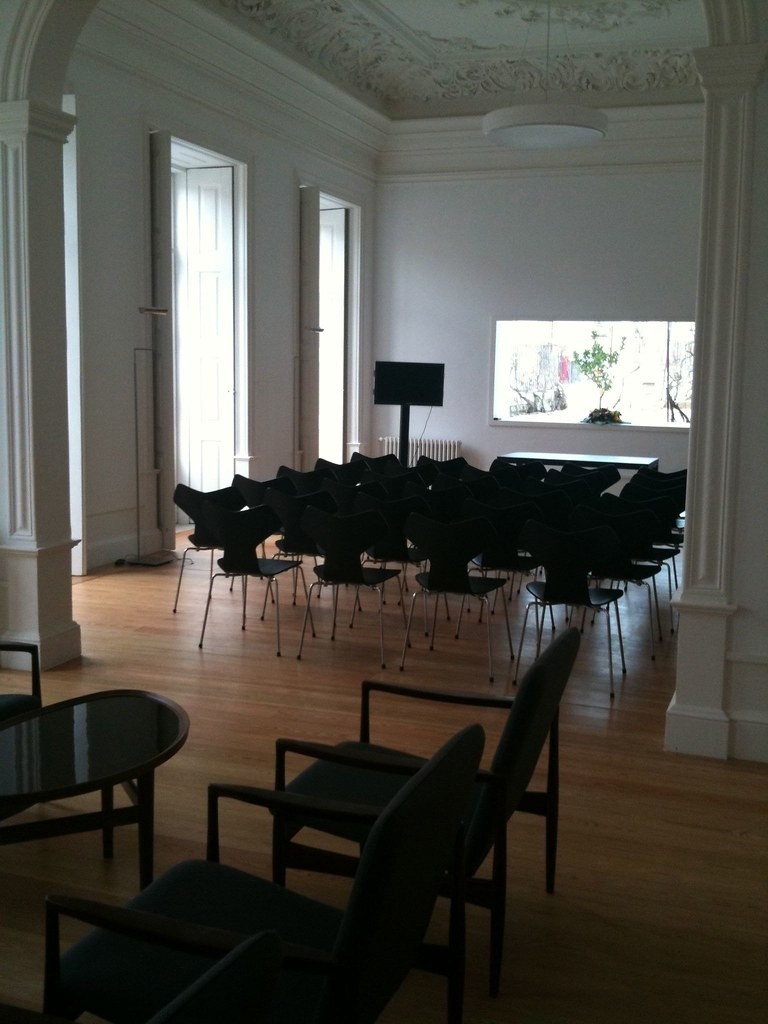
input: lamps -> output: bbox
[373,358,445,470]
[481,0,608,150]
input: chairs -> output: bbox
[43,724,486,1024]
[0,639,48,804]
[268,625,580,1001]
[171,450,687,701]
[0,930,283,1024]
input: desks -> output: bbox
[498,449,660,472]
[0,689,190,892]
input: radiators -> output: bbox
[377,436,463,468]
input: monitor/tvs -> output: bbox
[372,360,445,410]
